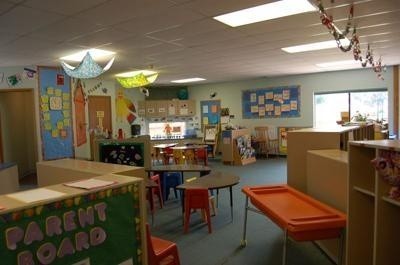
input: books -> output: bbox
[235,133,255,160]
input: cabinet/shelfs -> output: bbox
[302,145,349,265]
[34,158,146,218]
[0,174,148,265]
[345,139,399,265]
[137,98,198,118]
[220,126,257,166]
[282,121,373,199]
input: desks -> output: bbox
[174,169,241,216]
[151,164,212,179]
[150,137,219,168]
[238,181,348,264]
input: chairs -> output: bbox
[145,172,164,213]
[181,185,213,235]
[257,129,280,162]
[184,176,217,223]
[146,223,180,265]
